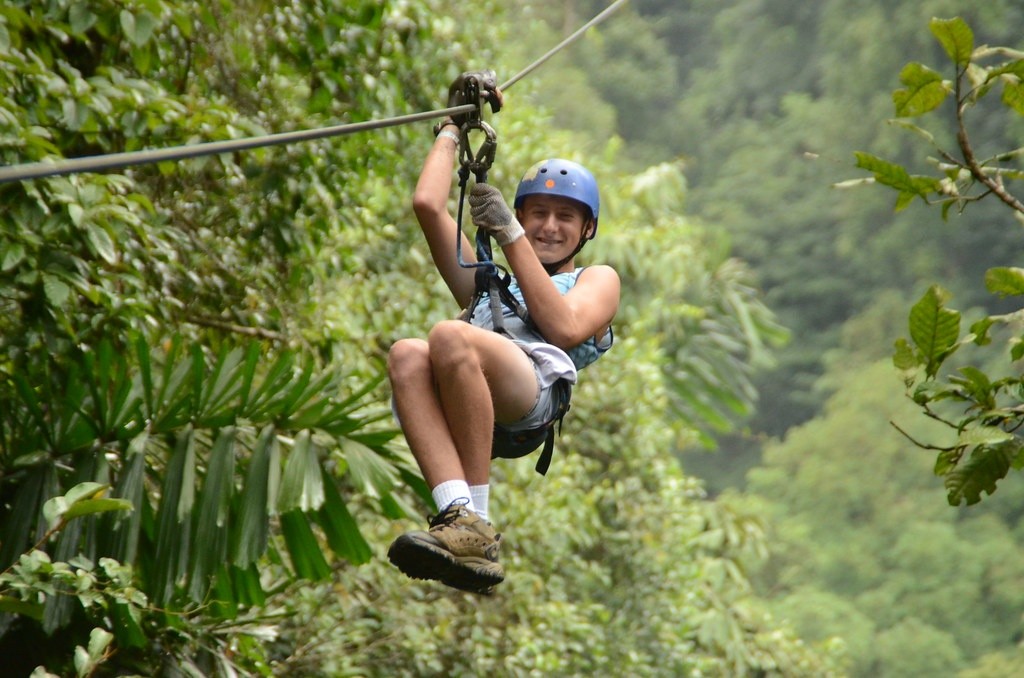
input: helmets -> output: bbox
[512,158,601,221]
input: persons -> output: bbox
[386,71,621,596]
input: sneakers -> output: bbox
[386,506,505,593]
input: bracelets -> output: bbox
[433,119,456,137]
[433,130,459,145]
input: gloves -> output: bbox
[468,182,525,247]
